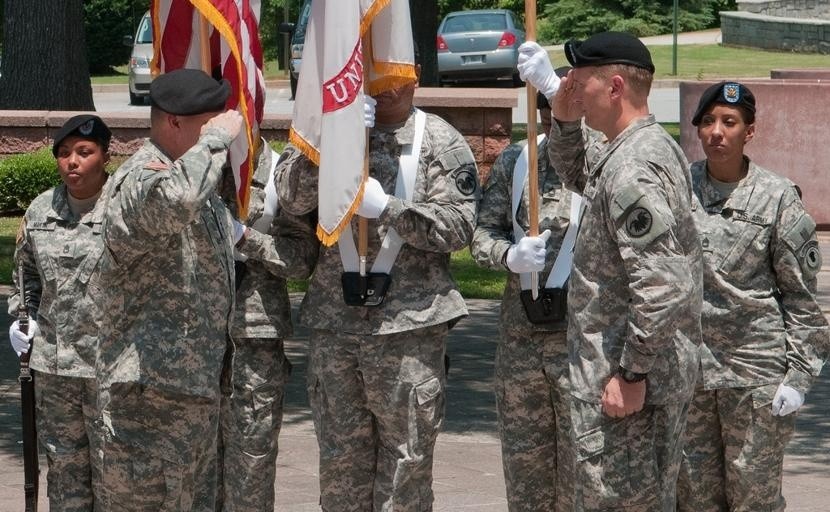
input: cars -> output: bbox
[437,7,533,84]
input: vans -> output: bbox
[121,7,173,108]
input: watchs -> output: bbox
[620,364,653,393]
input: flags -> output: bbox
[145,2,266,220]
[289,0,417,249]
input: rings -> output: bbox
[563,85,572,93]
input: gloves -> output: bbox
[506,229,552,273]
[364,94,377,128]
[516,40,561,100]
[355,176,390,219]
[772,383,806,417]
[9,314,37,357]
[231,216,246,246]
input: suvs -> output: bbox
[278,2,327,103]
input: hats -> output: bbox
[537,66,573,109]
[564,31,655,75]
[54,114,111,160]
[692,82,756,126]
[149,69,232,116]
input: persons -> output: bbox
[545,28,705,509]
[468,68,608,511]
[271,38,485,511]
[6,115,116,512]
[674,77,828,511]
[212,114,293,512]
[95,63,247,511]
